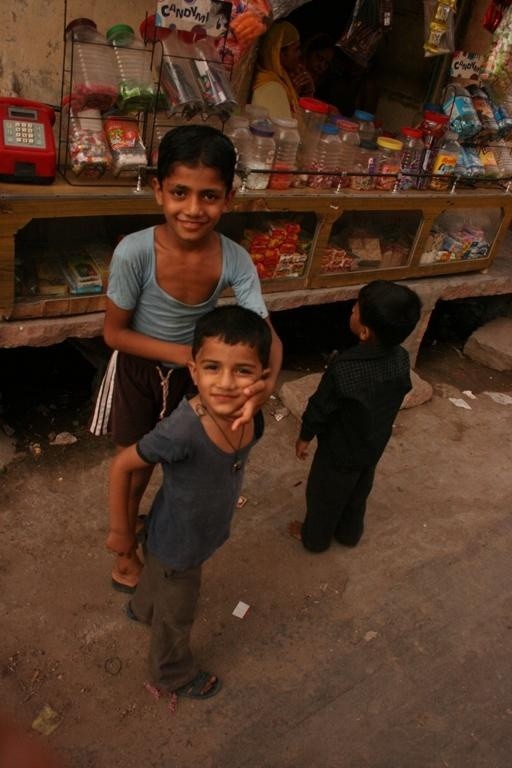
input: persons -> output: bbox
[103,303,272,701]
[287,279,424,555]
[91,124,284,594]
[249,19,315,120]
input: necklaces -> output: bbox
[194,393,245,475]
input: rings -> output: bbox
[304,71,307,76]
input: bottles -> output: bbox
[61,13,512,188]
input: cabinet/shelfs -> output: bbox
[0,162,512,324]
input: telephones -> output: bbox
[0,95,58,187]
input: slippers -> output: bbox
[111,577,137,593]
[177,669,221,698]
